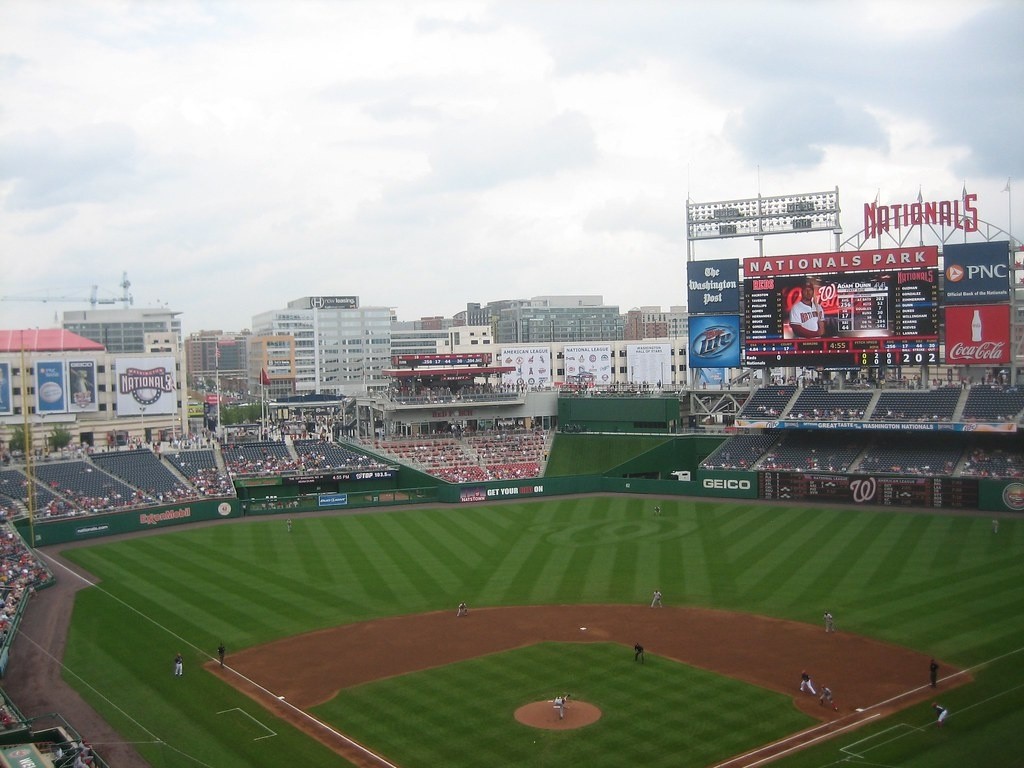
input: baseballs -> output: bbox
[39,382,62,403]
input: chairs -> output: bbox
[293,439,374,472]
[784,386,874,421]
[361,438,489,484]
[0,470,86,523]
[467,433,544,480]
[739,386,798,420]
[23,461,159,516]
[869,387,963,422]
[88,448,199,503]
[0,526,41,602]
[221,440,297,493]
[706,432,782,470]
[755,431,876,473]
[855,435,970,476]
[164,449,219,485]
[960,434,1024,478]
[960,385,1024,421]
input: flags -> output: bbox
[215,339,222,359]
[260,366,271,386]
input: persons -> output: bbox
[633,641,645,664]
[821,611,835,634]
[574,368,1023,482]
[932,701,947,730]
[799,669,816,696]
[650,588,663,609]
[818,684,838,711]
[0,419,549,658]
[928,658,939,688]
[789,283,824,353]
[553,694,571,710]
[69,738,98,768]
[174,653,183,678]
[216,643,226,668]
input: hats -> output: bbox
[801,282,813,290]
